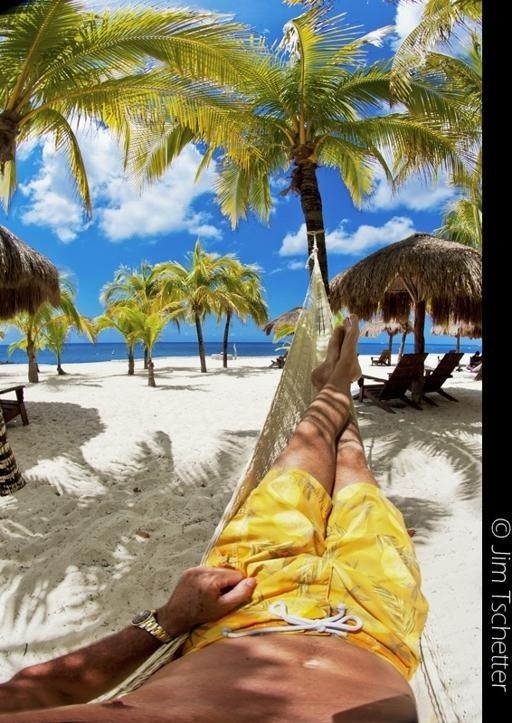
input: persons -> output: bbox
[1,311,433,723]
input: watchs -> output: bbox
[130,608,173,642]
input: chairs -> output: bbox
[0,384,30,425]
[423,353,464,407]
[371,350,390,366]
[352,352,428,414]
